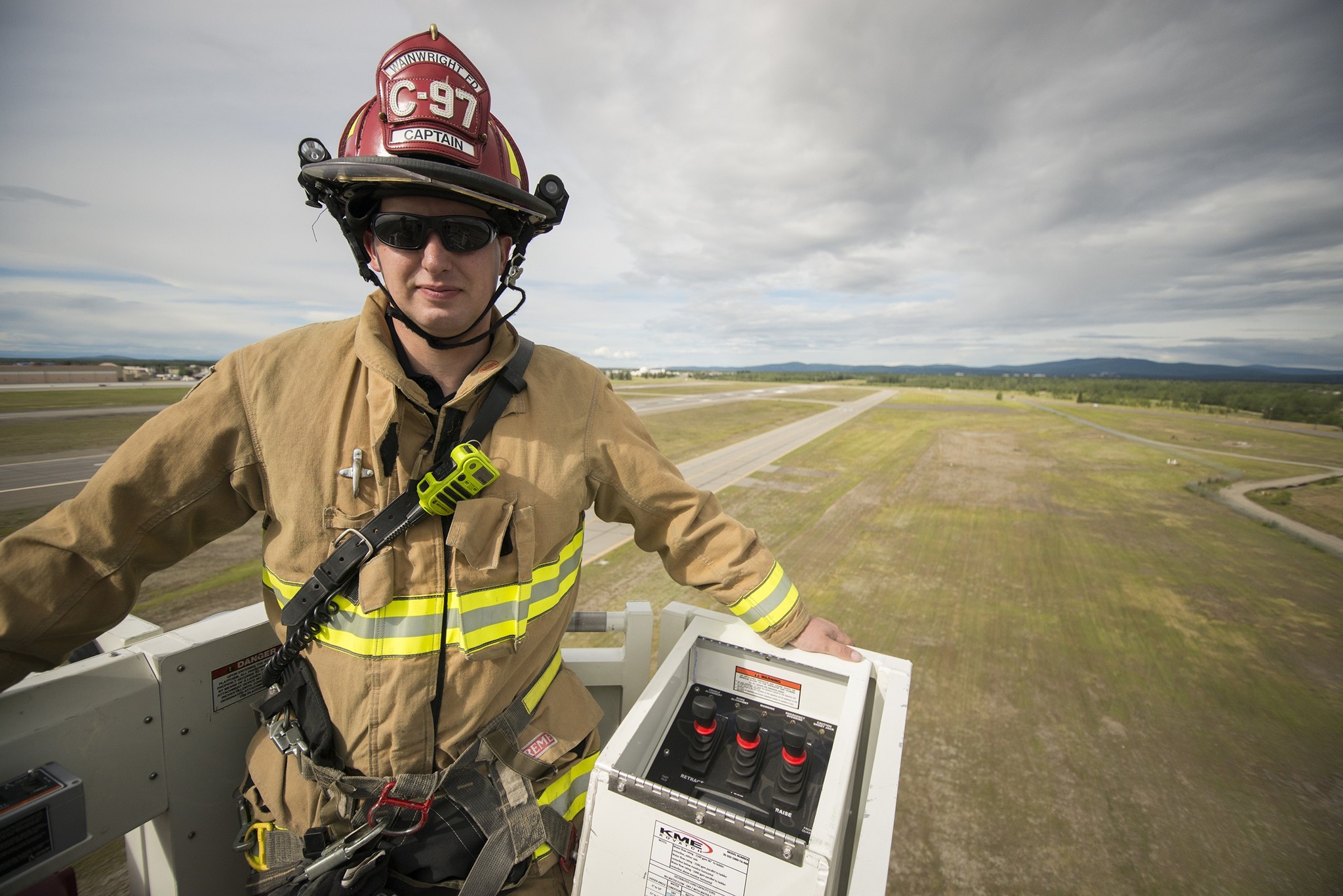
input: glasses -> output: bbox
[367,211,500,253]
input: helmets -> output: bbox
[297,23,570,237]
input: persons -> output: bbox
[1,24,859,895]
